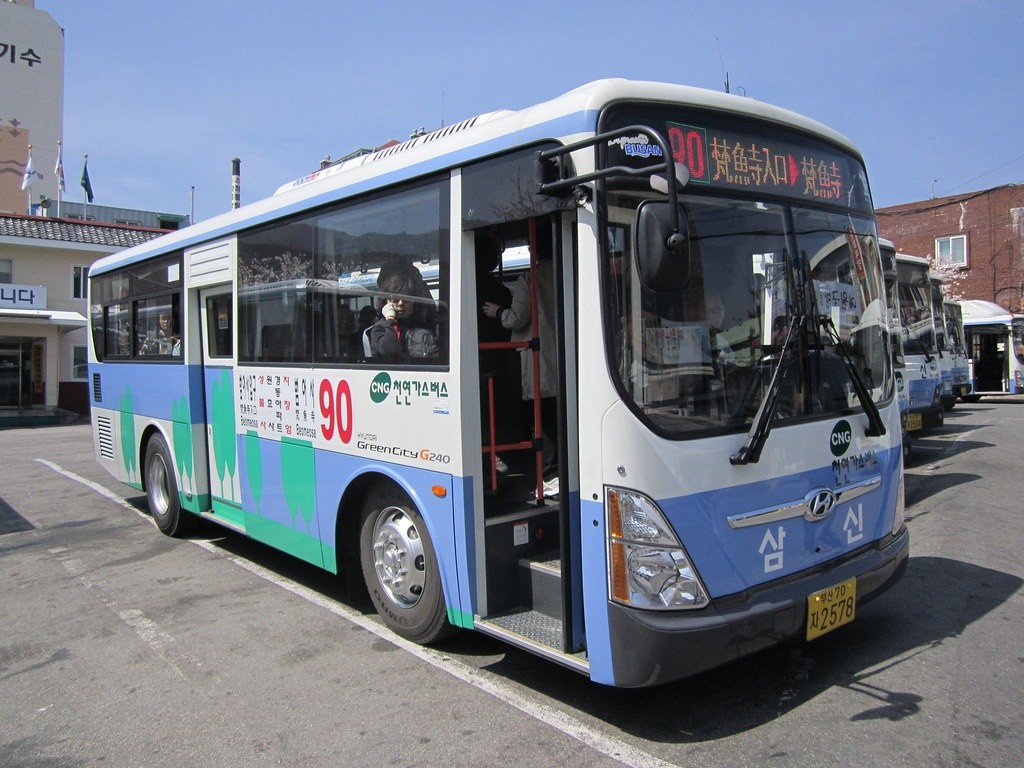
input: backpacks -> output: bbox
[397,319,440,365]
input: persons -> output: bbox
[348,305,377,357]
[474,226,514,344]
[482,224,563,474]
[118,310,180,356]
[370,272,423,362]
[377,262,438,339]
[694,288,737,334]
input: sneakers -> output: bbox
[535,478,559,499]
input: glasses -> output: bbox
[698,306,723,315]
[387,296,409,303]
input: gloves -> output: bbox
[382,303,397,319]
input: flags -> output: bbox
[54,156,65,192]
[80,162,94,203]
[21,157,41,191]
